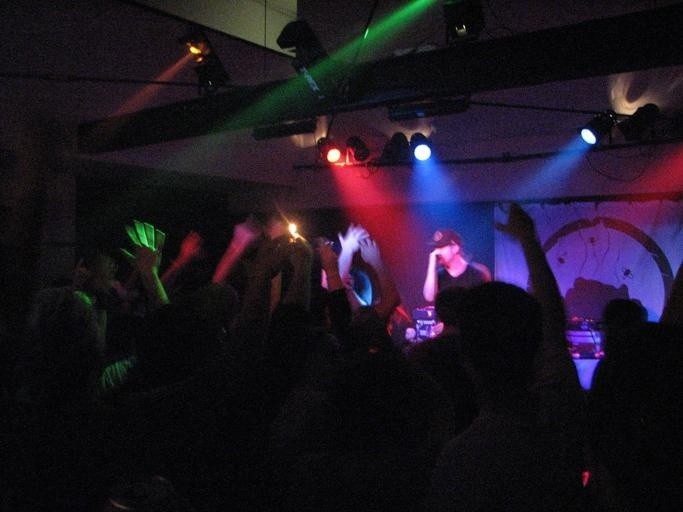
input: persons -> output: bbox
[2,201,683,511]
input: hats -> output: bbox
[427,229,462,247]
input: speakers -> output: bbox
[75,158,298,256]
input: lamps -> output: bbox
[442,2,486,47]
[275,19,342,101]
[316,130,432,168]
[580,102,660,147]
[178,27,229,93]
